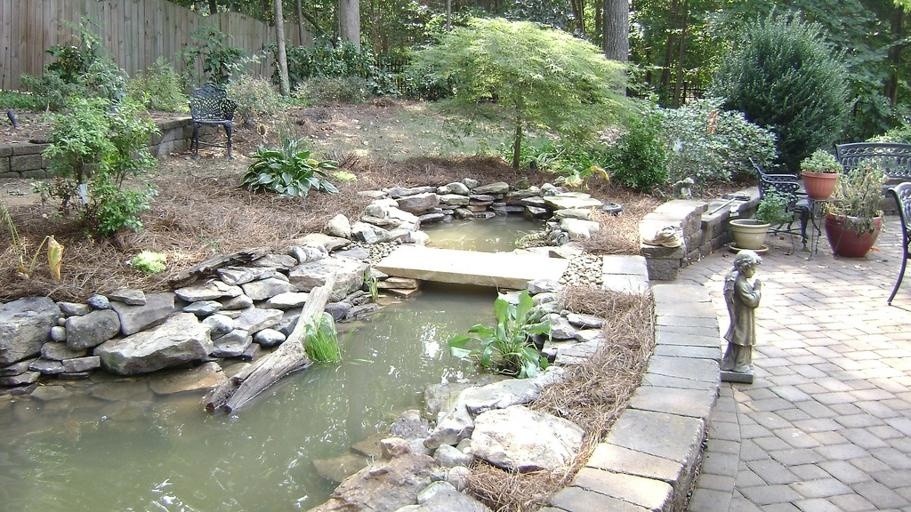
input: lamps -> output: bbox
[888,182,911,307]
[749,155,823,250]
[190,84,239,158]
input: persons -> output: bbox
[718,248,762,373]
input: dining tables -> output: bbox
[825,158,889,258]
[727,195,791,252]
[802,148,843,200]
